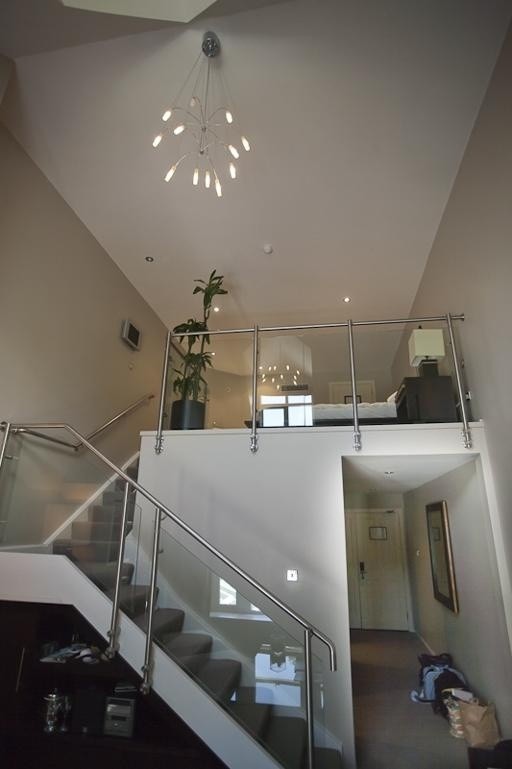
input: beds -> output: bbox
[312,402,399,426]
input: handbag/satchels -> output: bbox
[410,654,502,751]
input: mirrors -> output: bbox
[425,501,458,614]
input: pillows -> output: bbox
[386,390,398,402]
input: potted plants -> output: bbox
[167,269,232,430]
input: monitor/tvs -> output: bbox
[122,318,142,349]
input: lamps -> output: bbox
[152,30,251,197]
[407,327,446,377]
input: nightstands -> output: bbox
[394,377,457,423]
